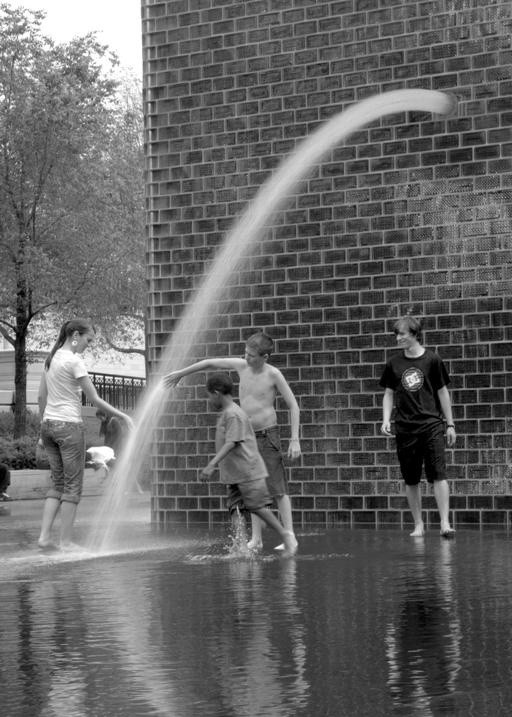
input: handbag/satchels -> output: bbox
[38,437,45,447]
[447,425,456,428]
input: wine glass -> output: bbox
[381,431,384,432]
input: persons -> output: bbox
[0,463,11,501]
[162,332,302,551]
[379,316,458,537]
[199,373,298,559]
[95,407,145,495]
[35,319,135,552]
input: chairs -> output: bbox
[1,492,11,500]
